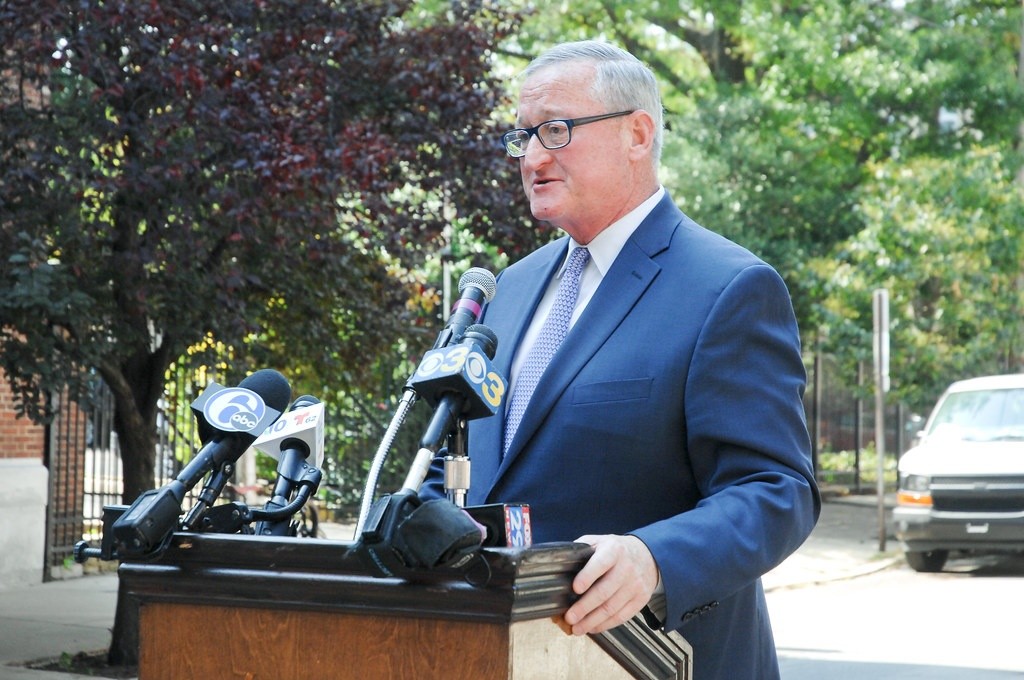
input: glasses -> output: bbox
[500,111,634,158]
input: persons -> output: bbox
[412,41,821,680]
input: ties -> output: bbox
[501,247,590,462]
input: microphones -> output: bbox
[112,370,325,555]
[352,267,531,572]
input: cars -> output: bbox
[890,372,1024,572]
